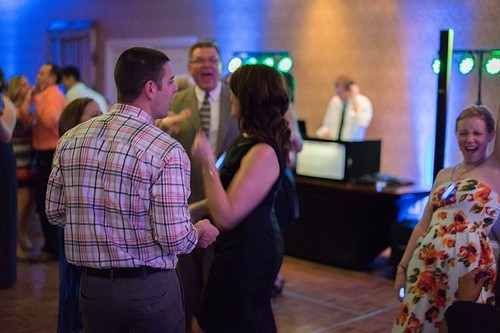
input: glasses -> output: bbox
[188,57,218,64]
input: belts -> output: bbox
[80,266,170,280]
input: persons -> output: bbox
[149,40,244,333]
[315,75,374,140]
[56,96,192,333]
[187,64,304,333]
[393,105,500,333]
[443,254,500,333]
[45,47,219,333]
[0,63,108,292]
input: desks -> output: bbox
[283,177,413,268]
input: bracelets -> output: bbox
[396,263,407,274]
[199,165,218,179]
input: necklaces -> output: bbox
[458,158,483,174]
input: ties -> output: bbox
[200,92,212,138]
[338,103,348,141]
